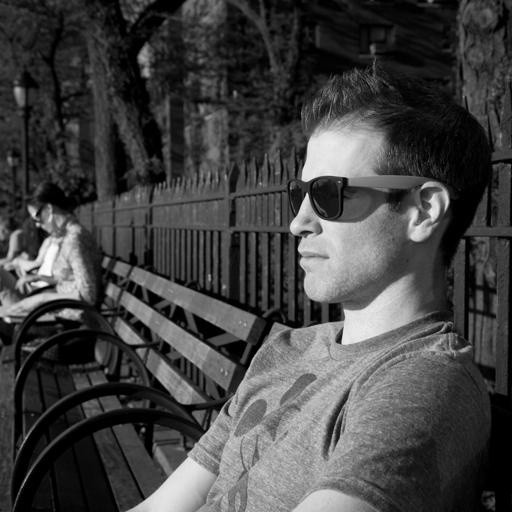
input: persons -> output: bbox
[0,180,100,348]
[116,60,499,511]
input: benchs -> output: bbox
[7,256,133,366]
[8,266,262,510]
[7,308,512,510]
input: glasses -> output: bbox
[287,175,437,220]
[32,205,44,222]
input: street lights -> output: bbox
[13,63,40,220]
[7,150,22,214]
